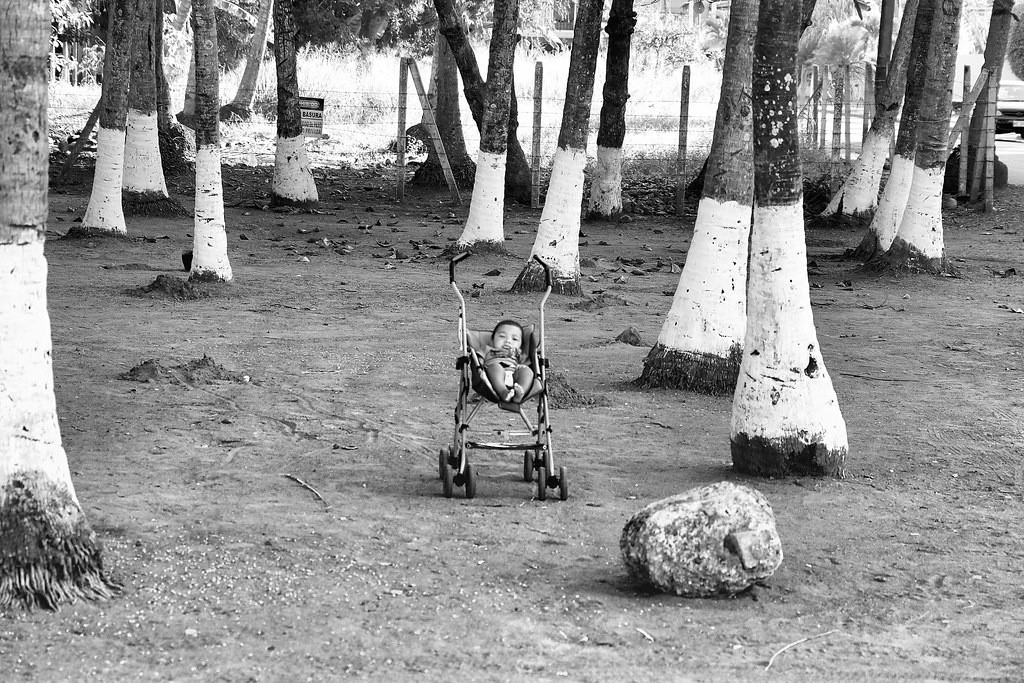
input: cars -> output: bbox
[995,79,1024,139]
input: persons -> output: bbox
[476,320,534,403]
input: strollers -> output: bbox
[438,250,568,501]
